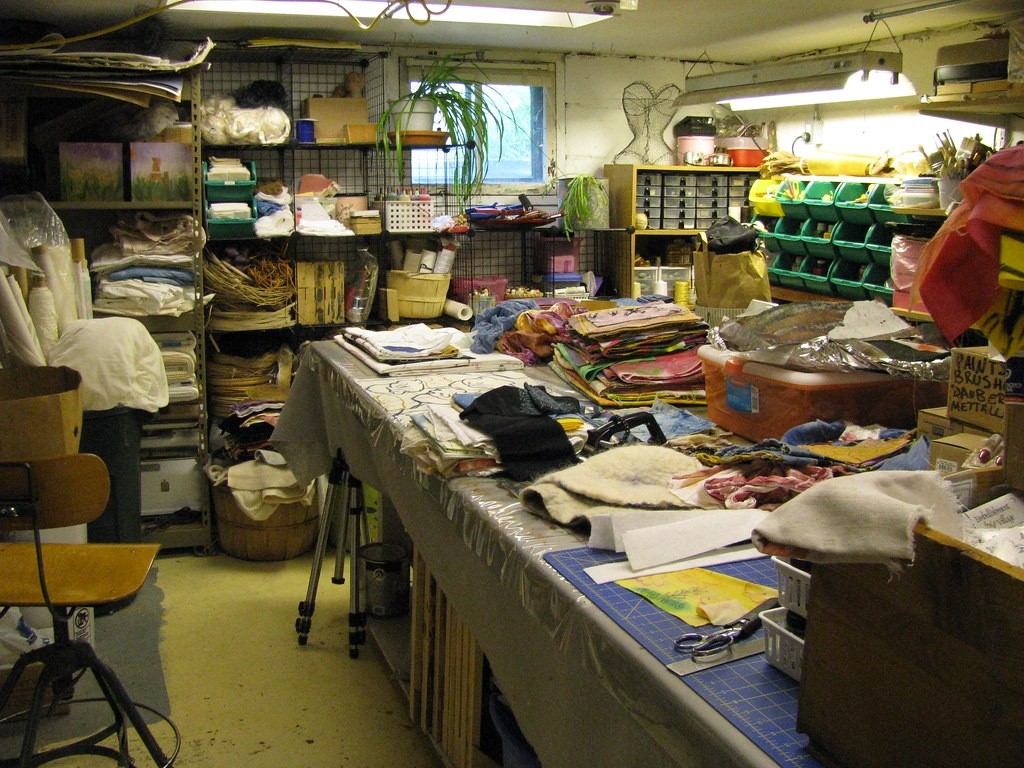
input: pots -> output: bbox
[707,152,730,166]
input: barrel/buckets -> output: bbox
[557,176,609,229]
[676,136,715,166]
[938,180,962,211]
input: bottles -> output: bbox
[467,287,495,314]
[374,187,431,232]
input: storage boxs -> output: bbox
[1,99,34,195]
[532,273,589,300]
[378,288,398,323]
[698,343,953,445]
[635,237,696,302]
[636,173,759,229]
[486,675,540,768]
[673,115,766,169]
[0,364,84,458]
[342,122,377,145]
[534,236,583,273]
[757,467,1024,768]
[916,345,1023,540]
[303,97,369,144]
[370,198,433,233]
[747,177,932,304]
[451,275,508,316]
[202,161,257,238]
[0,663,72,723]
[158,403,199,420]
[140,458,202,516]
[45,141,124,203]
[125,139,192,202]
[932,37,1008,97]
[890,233,934,314]
[297,260,346,326]
[290,173,382,235]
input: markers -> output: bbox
[979,434,1004,467]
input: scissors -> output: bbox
[673,596,779,656]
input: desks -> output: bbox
[268,338,820,768]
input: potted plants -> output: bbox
[375,52,517,217]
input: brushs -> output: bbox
[928,132,990,180]
[472,194,533,215]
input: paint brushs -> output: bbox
[919,128,958,171]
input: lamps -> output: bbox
[669,19,918,113]
[155,0,620,29]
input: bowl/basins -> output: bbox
[724,149,769,166]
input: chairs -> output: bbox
[1,453,181,768]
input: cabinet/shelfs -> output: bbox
[884,74,1024,329]
[603,163,763,298]
[378,141,475,325]
[457,217,636,300]
[195,37,386,552]
[0,43,203,551]
